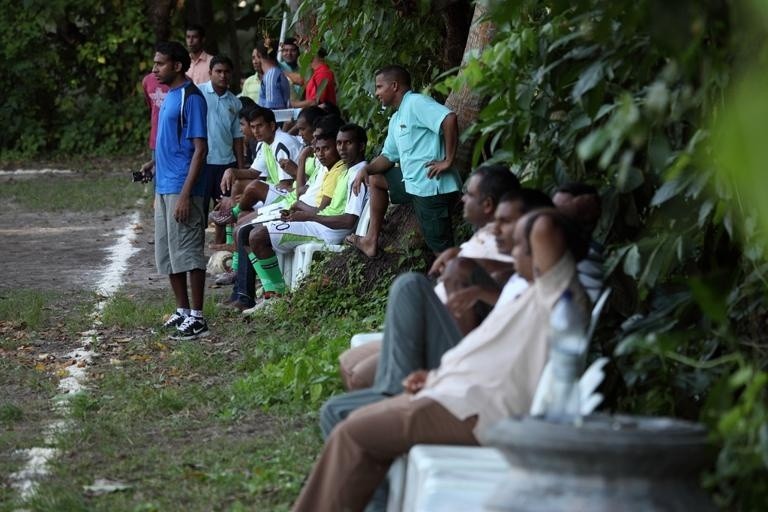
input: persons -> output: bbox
[139,22,372,339]
[288,164,605,511]
[345,66,462,259]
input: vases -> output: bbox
[465,409,716,511]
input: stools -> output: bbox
[275,244,294,288]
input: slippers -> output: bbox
[344,235,384,260]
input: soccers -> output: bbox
[207,248,234,280]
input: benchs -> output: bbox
[347,242,621,511]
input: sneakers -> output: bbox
[215,296,256,312]
[209,208,238,226]
[169,315,212,342]
[215,270,240,285]
[240,289,294,320]
[158,311,189,330]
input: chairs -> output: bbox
[289,190,377,296]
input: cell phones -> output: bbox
[132,170,152,182]
[280,209,294,215]
